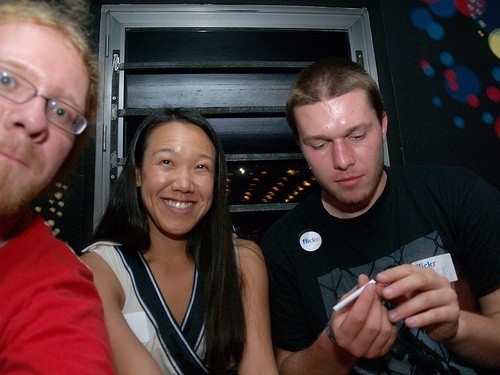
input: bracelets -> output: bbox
[325,321,338,345]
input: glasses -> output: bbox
[0,67,88,135]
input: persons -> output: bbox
[0,0,116,375]
[258,57,500,375]
[79,106,279,375]
